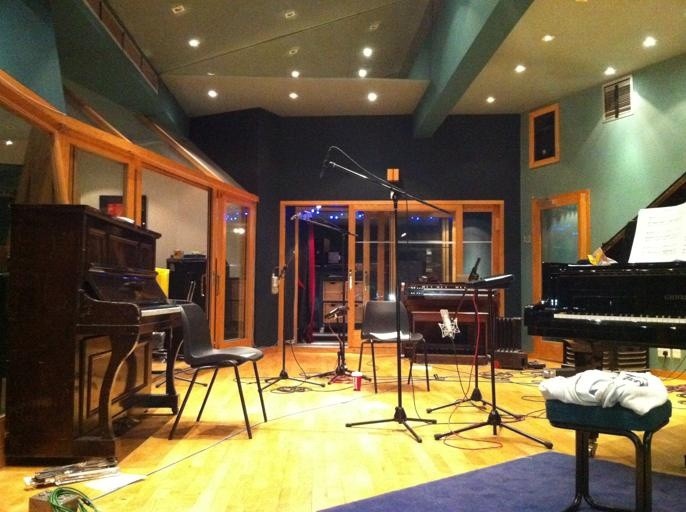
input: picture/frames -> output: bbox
[527,103,561,170]
[601,74,635,125]
[99,194,147,229]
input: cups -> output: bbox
[351,372,363,391]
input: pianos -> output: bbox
[399,282,500,365]
[0,205,189,468]
[524,261,686,378]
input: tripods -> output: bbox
[426,257,523,418]
[330,162,452,443]
[434,289,554,450]
[258,251,326,392]
[302,218,372,385]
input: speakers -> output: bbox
[344,280,363,302]
[323,302,345,323]
[345,302,363,324]
[323,280,344,302]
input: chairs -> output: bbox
[358,300,431,396]
[169,303,268,444]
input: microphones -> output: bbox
[271,272,279,294]
[324,305,346,318]
[439,308,454,341]
[482,273,514,287]
[290,211,306,221]
[320,148,332,181]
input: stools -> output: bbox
[545,398,672,512]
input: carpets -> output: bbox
[317,450,686,512]
